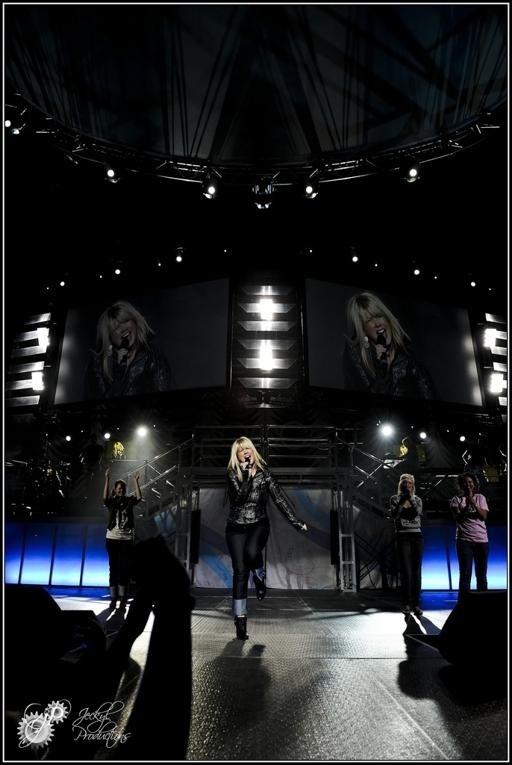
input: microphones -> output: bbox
[376,334,388,366]
[119,339,129,366]
[244,459,250,477]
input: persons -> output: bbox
[85,299,180,396]
[446,470,492,597]
[345,291,438,398]
[223,435,309,642]
[386,471,427,616]
[6,557,197,762]
[101,466,142,608]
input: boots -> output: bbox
[234,615,249,639]
[252,574,266,601]
